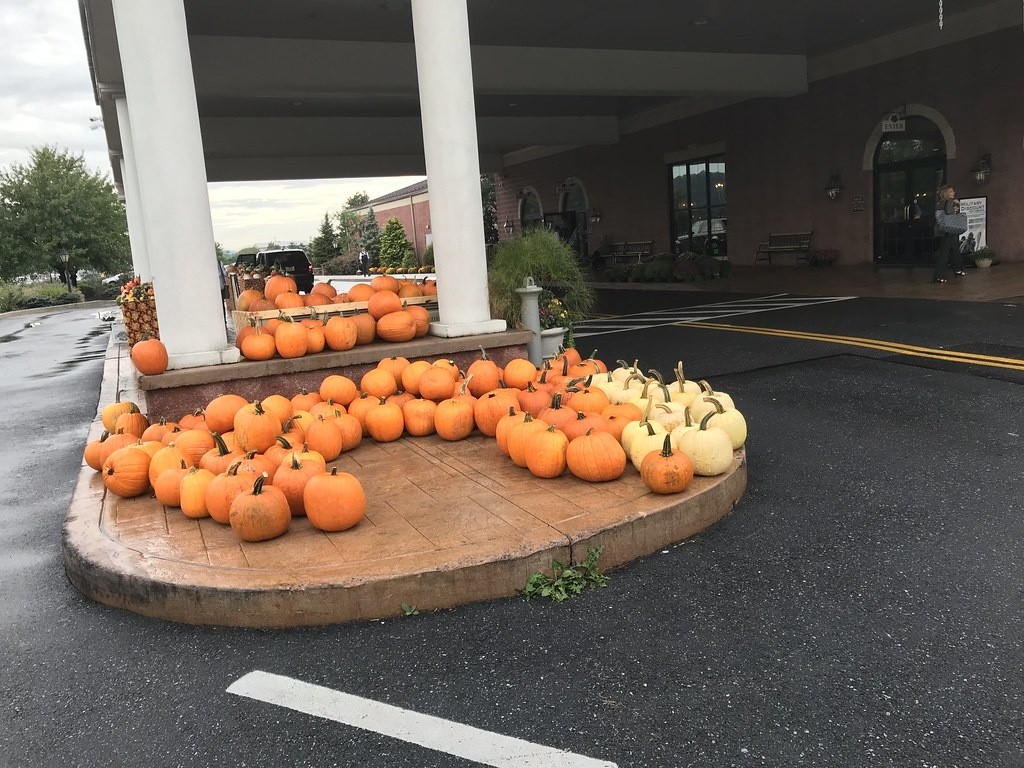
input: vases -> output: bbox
[628,276,634,282]
[640,277,647,283]
[544,327,568,363]
[818,258,831,267]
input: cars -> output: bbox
[101,272,124,286]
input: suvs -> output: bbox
[233,249,315,295]
[676,218,728,255]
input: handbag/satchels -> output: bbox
[222,285,229,299]
[935,199,967,235]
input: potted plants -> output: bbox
[974,247,996,268]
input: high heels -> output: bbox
[933,278,947,284]
[954,269,967,278]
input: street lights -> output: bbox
[60,247,72,292]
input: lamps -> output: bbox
[970,147,991,183]
[503,219,514,233]
[588,208,601,227]
[825,177,840,200]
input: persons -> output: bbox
[935,185,967,283]
[359,248,371,277]
[959,232,976,254]
[218,261,228,329]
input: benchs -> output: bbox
[754,230,816,268]
[590,240,655,267]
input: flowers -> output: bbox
[540,299,569,329]
[816,249,839,259]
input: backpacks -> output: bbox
[361,251,368,264]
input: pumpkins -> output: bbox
[83,343,747,543]
[119,264,438,375]
